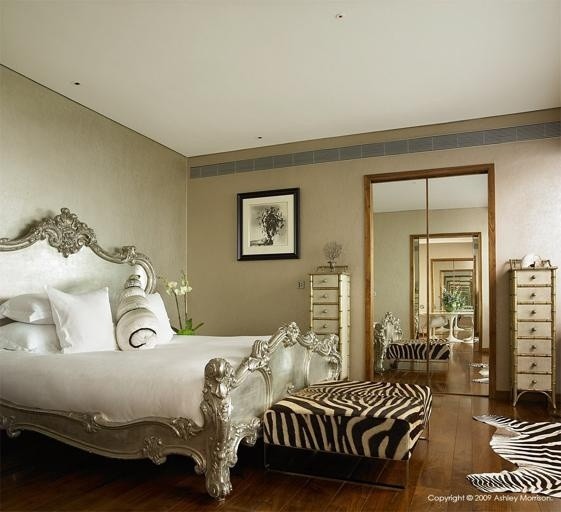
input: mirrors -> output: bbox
[361,162,499,400]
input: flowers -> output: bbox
[441,288,467,309]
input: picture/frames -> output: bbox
[235,186,302,263]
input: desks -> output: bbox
[427,312,475,343]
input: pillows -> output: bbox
[0,285,178,356]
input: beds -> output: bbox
[372,312,407,376]
[0,205,345,502]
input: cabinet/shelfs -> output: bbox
[308,265,351,382]
[509,257,559,410]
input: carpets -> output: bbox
[465,410,561,499]
[469,361,490,385]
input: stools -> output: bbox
[387,335,454,377]
[258,375,434,493]
